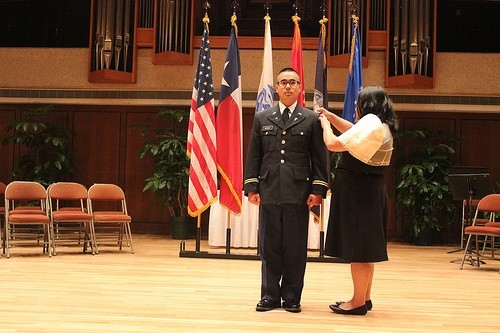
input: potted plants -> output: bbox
[391,142,456,245]
[139,107,195,240]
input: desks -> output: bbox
[447,173,490,266]
[207,190,331,250]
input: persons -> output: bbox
[243,68,329,312]
[317,85,398,314]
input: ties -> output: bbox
[282,108,290,126]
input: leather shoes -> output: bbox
[283,298,301,311]
[255,298,281,310]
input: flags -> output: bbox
[216,17,245,216]
[187,17,218,217]
[310,17,328,224]
[292,16,305,108]
[254,16,274,114]
[339,17,362,160]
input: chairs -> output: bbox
[0,181,135,258]
[460,194,500,274]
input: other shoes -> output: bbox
[329,303,367,315]
[336,300,372,310]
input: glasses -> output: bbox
[277,79,300,88]
